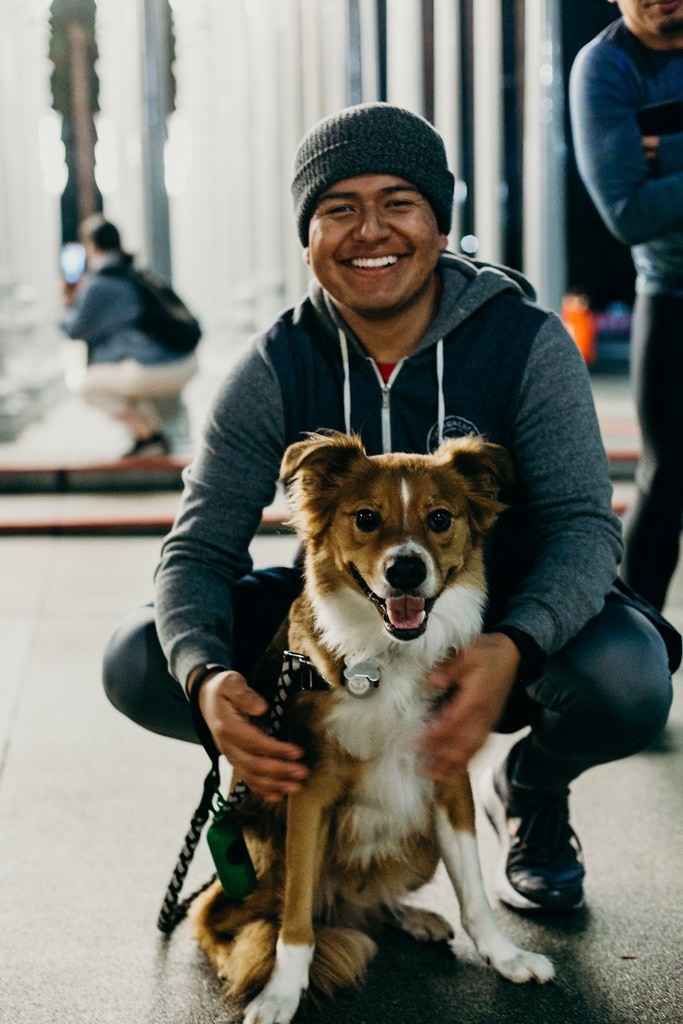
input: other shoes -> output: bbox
[122,431,170,459]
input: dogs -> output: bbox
[193,427,556,1024]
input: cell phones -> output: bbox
[60,243,86,285]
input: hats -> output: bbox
[291,100,455,247]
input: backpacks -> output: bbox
[98,266,201,356]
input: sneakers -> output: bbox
[481,736,587,909]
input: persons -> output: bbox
[97,103,673,916]
[57,216,196,460]
[568,0,683,615]
